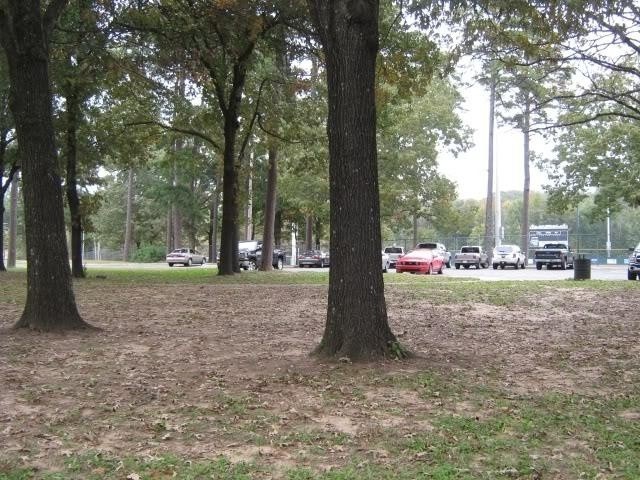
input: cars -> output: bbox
[627,242,640,280]
[216,239,286,272]
[491,244,526,270]
[165,248,207,267]
[381,243,452,276]
[298,249,330,268]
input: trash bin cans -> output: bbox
[575,259,592,279]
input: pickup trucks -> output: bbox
[534,243,575,270]
[454,245,490,269]
